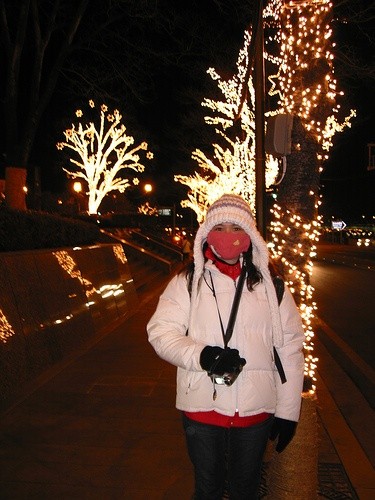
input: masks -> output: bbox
[208,230,250,260]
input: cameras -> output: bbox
[211,358,247,386]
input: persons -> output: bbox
[146,194,305,500]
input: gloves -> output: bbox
[269,417,298,452]
[200,346,246,376]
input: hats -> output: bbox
[188,194,284,349]
